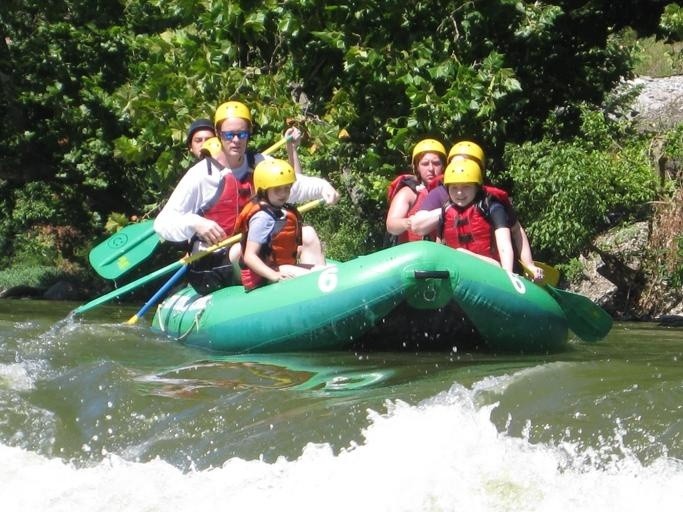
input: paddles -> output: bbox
[518,260,560,287]
[89,135,293,281]
[515,257,615,342]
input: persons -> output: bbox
[297,223,323,268]
[235,158,302,282]
[412,140,544,281]
[185,119,302,176]
[386,138,447,243]
[154,100,339,292]
[442,159,515,272]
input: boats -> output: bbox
[154,239,570,353]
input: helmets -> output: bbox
[186,99,250,162]
[412,136,487,191]
[253,159,297,194]
